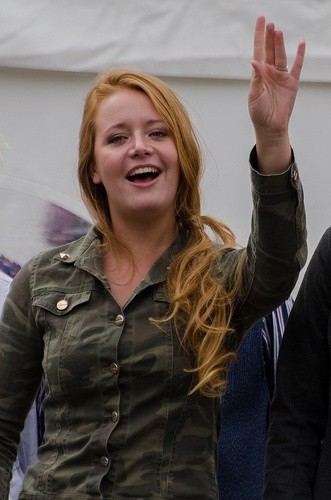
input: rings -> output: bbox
[274,64,289,71]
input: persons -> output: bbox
[266,227,329,500]
[2,15,312,499]
